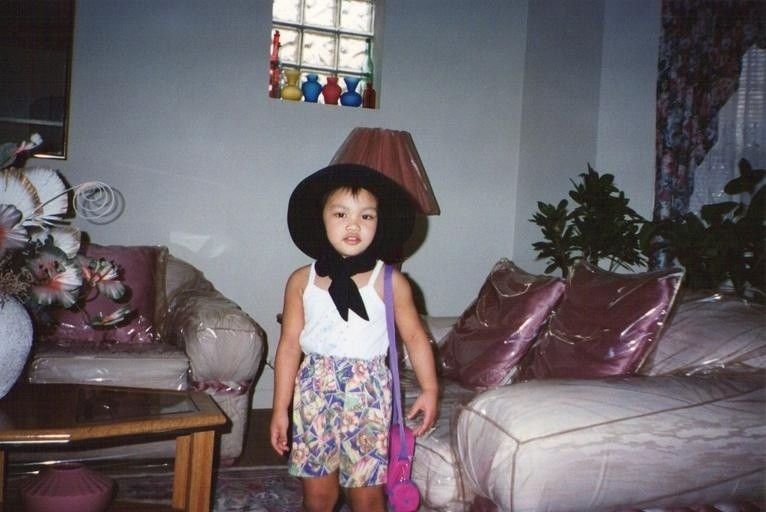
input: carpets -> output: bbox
[21,453,356,511]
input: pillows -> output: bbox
[33,239,169,350]
[427,252,688,404]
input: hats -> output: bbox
[287,163,418,265]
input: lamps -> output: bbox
[330,125,442,219]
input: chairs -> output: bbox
[0,233,268,469]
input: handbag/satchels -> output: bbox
[383,424,415,496]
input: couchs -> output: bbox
[376,285,766,511]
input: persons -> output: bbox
[268,164,439,512]
